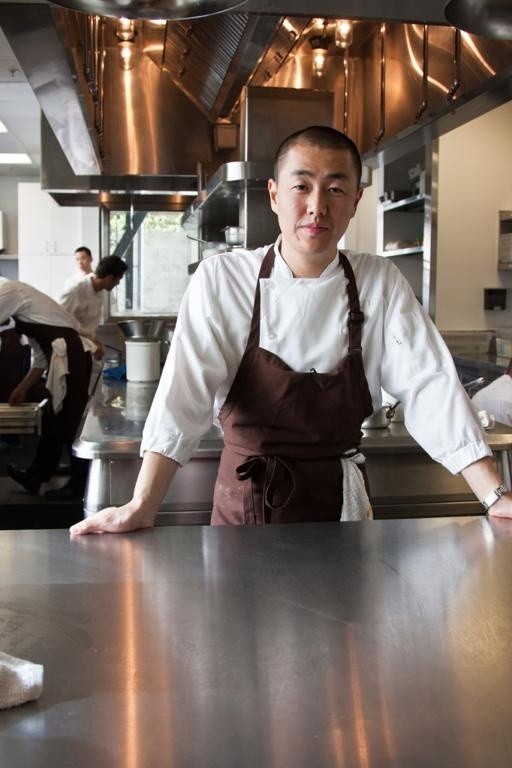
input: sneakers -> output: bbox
[1,474,44,508]
[40,474,71,495]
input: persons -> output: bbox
[66,123,512,534]
[51,252,130,336]
[74,243,93,274]
[0,271,97,454]
[470,355,512,492]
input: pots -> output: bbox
[186,233,226,260]
[221,224,244,244]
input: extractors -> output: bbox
[0,0,512,179]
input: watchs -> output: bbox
[480,481,508,511]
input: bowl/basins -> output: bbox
[118,319,165,340]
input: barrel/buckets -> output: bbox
[125,381,158,422]
